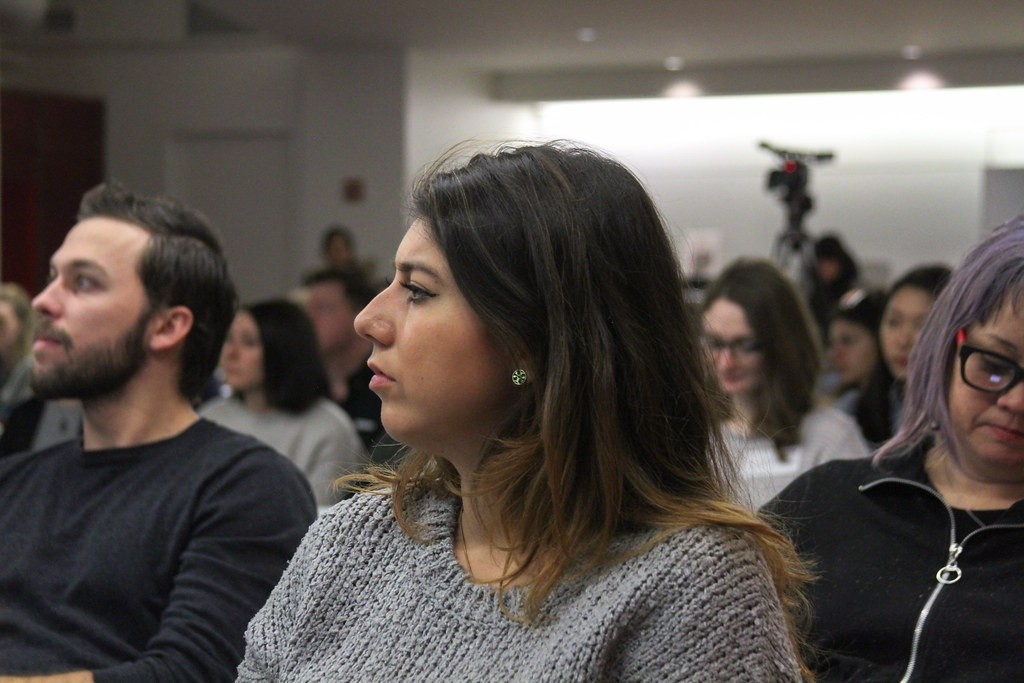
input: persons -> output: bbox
[0,145,1024,683]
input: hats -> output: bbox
[827,286,888,329]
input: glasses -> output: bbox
[695,331,765,354]
[954,328,1024,395]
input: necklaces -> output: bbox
[949,480,985,527]
[455,504,478,581]
